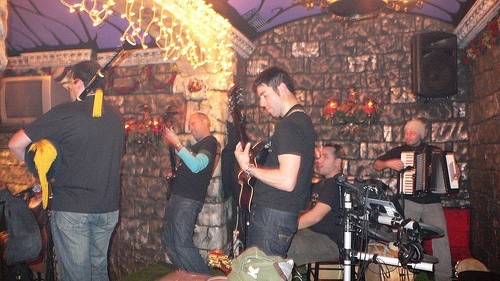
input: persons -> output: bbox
[7,60,128,281]
[287,143,347,267]
[374,118,460,281]
[163,113,218,276]
[235,67,316,258]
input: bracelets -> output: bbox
[245,164,255,177]
[174,142,182,147]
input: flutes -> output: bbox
[72,32,137,104]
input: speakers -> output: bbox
[410,30,459,98]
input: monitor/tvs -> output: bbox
[0,75,69,125]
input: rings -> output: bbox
[399,165,402,167]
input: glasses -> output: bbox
[66,77,80,92]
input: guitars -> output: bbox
[227,85,269,214]
[166,127,182,201]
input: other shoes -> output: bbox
[177,272,211,281]
[157,268,186,281]
